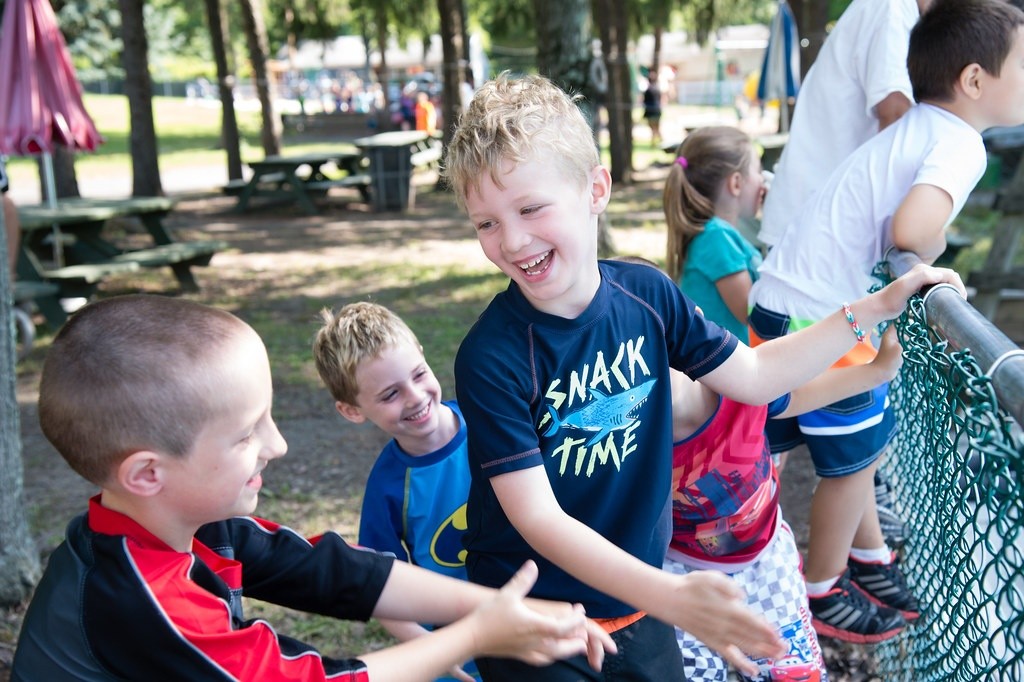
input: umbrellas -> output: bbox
[758,1,800,133]
[0,0,104,269]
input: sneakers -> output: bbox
[806,565,906,643]
[846,549,921,619]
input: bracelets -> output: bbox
[842,305,866,343]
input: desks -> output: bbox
[231,152,373,213]
[16,195,203,332]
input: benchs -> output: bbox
[217,170,301,194]
[340,166,374,187]
[11,238,229,304]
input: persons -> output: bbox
[663,125,770,348]
[395,83,436,136]
[453,74,967,682]
[0,167,19,290]
[9,295,618,682]
[644,71,663,149]
[313,302,483,681]
[756,0,931,476]
[748,0,1024,641]
[608,255,914,679]
